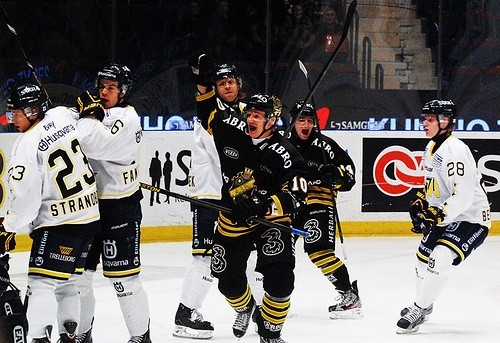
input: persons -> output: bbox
[0,0,364,343]
[396,99,492,333]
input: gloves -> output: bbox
[75,90,105,122]
[320,164,356,187]
[230,196,267,227]
[189,51,213,86]
[408,191,429,219]
[412,206,445,231]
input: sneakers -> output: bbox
[260,336,287,342]
[31,325,53,343]
[76,317,95,343]
[173,302,214,339]
[328,280,363,319]
[395,304,433,334]
[127,318,153,343]
[232,295,256,340]
[58,322,77,343]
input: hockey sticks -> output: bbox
[284,0,358,138]
[0,2,55,108]
[421,222,432,238]
[139,180,310,238]
[298,59,348,260]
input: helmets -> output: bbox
[95,63,134,100]
[419,98,457,123]
[6,84,50,123]
[242,93,282,124]
[290,100,317,128]
[214,63,240,87]
